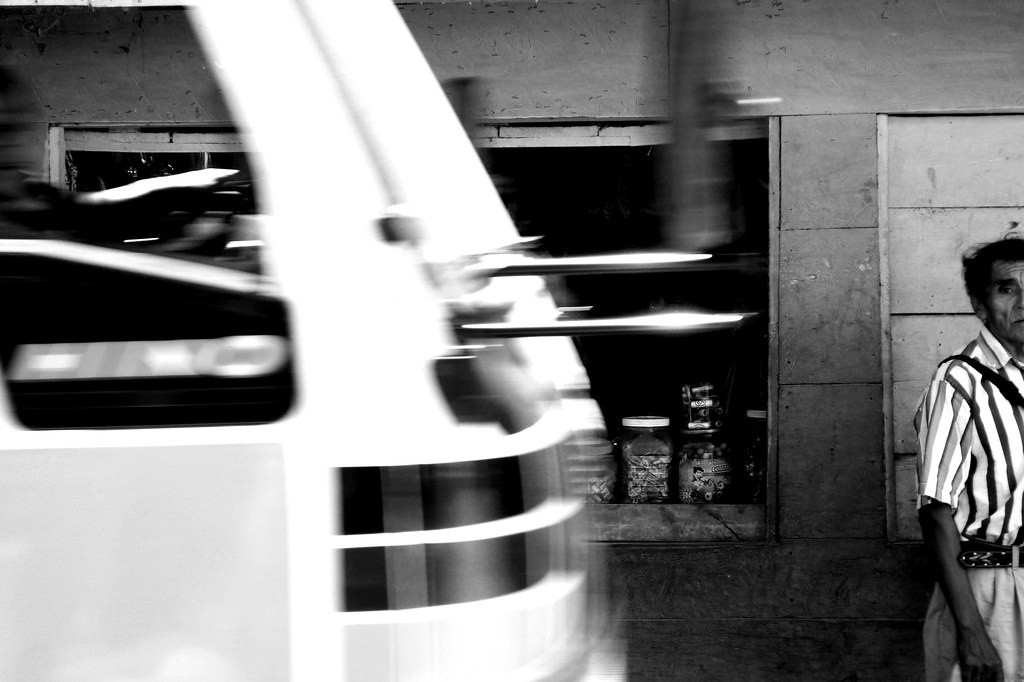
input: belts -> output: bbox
[958,541,1024,568]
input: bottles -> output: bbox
[622,415,674,504]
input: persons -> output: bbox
[913,238,1023,682]
[0,65,246,244]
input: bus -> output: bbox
[0,2,759,682]
